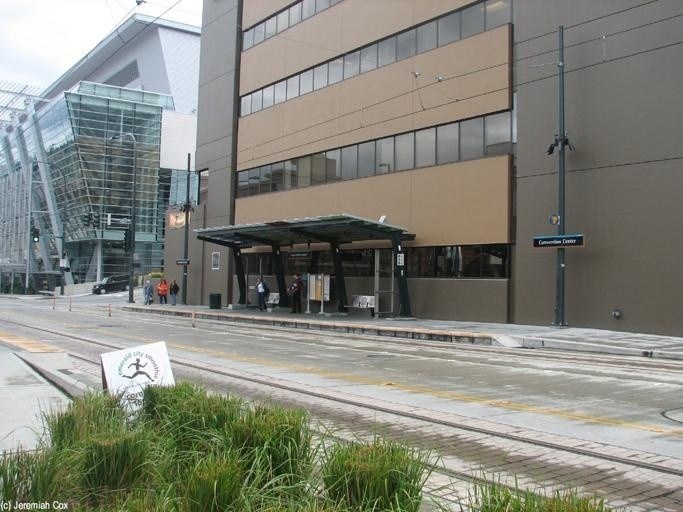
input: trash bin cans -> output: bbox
[209,293,221,309]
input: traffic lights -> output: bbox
[84,214,90,226]
[93,213,100,226]
[33,229,40,242]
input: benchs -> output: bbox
[265,292,280,305]
[342,294,375,316]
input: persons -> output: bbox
[290,273,302,313]
[143,279,179,306]
[255,277,269,311]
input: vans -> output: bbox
[93,274,130,294]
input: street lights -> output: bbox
[34,160,65,294]
[109,133,136,301]
